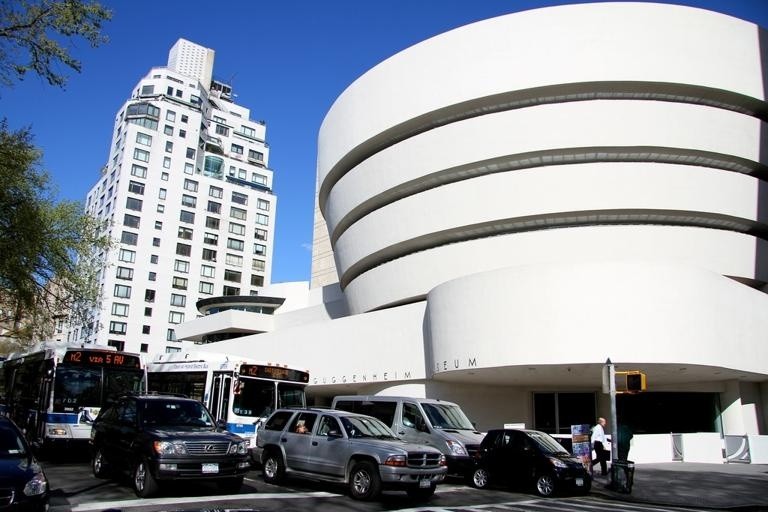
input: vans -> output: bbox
[330,396,485,478]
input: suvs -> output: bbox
[256,408,446,501]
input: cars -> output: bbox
[1,415,50,512]
[472,429,592,498]
[89,395,252,498]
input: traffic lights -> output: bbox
[626,374,646,390]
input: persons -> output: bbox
[588,418,608,476]
